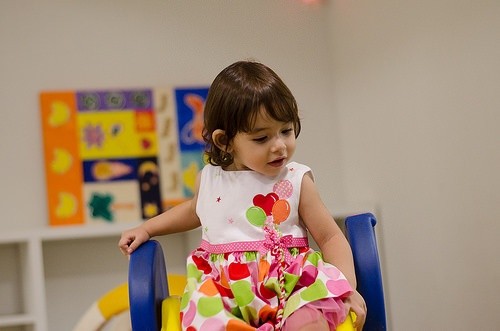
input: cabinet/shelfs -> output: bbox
[0,207,382,331]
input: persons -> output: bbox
[117,60,368,331]
